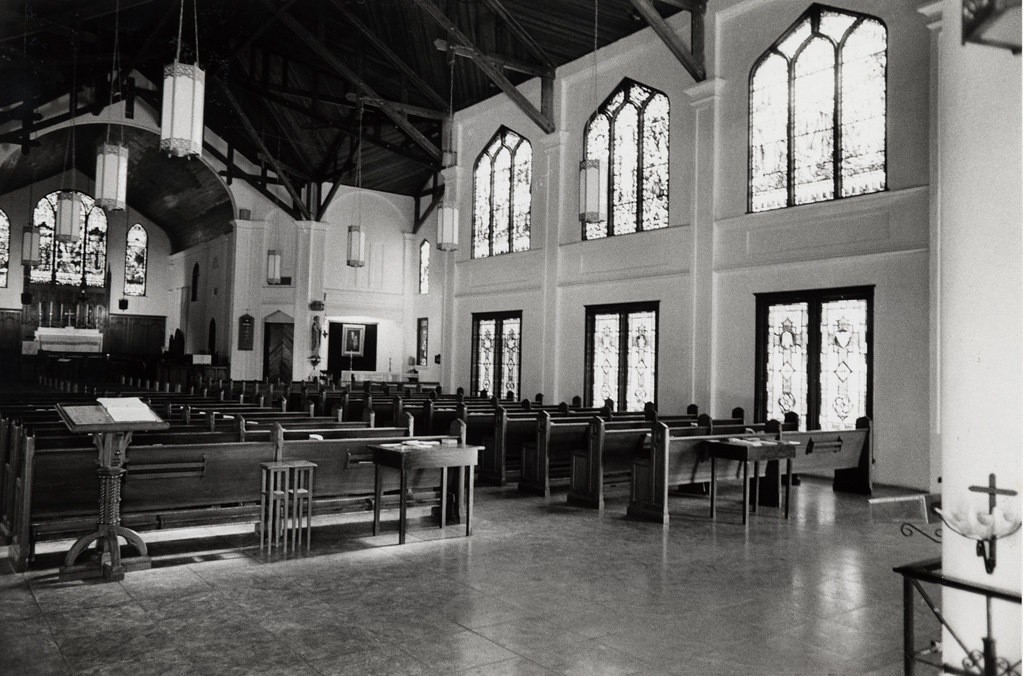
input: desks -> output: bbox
[366,442,485,545]
[341,370,399,383]
[705,439,802,525]
[35,327,104,352]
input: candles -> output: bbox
[38,301,99,326]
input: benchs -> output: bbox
[0,373,875,573]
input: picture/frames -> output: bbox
[341,324,366,357]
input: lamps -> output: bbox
[438,54,459,252]
[20,132,41,268]
[579,0,600,224]
[267,176,281,285]
[94,0,129,212]
[346,111,366,267]
[158,0,206,161]
[55,53,80,243]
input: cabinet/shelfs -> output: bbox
[103,313,168,354]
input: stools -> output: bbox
[259,460,318,556]
